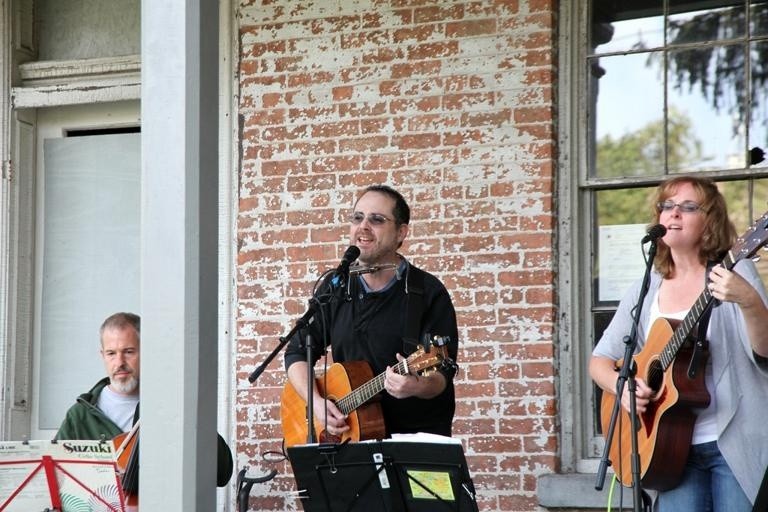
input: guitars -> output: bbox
[281,335,451,465]
[601,211,767,493]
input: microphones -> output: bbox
[640,223,666,244]
[338,245,360,275]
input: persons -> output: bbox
[587,175,768,512]
[53,313,233,488]
[284,186,458,439]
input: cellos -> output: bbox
[107,426,140,508]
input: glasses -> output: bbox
[347,213,399,225]
[657,201,711,214]
